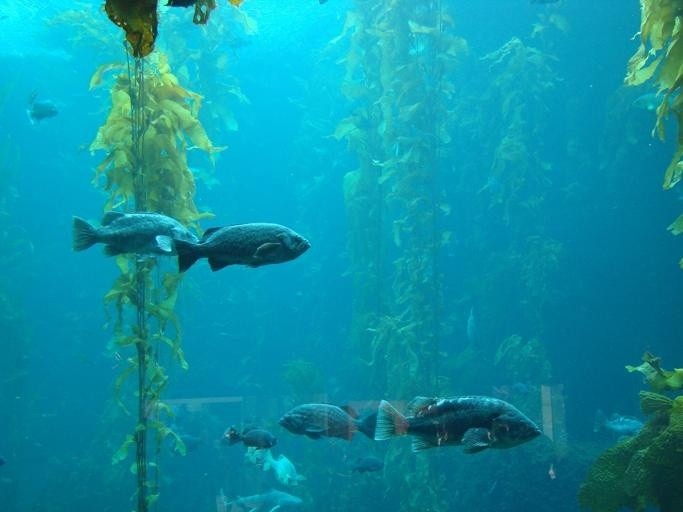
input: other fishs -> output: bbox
[26,100,59,126]
